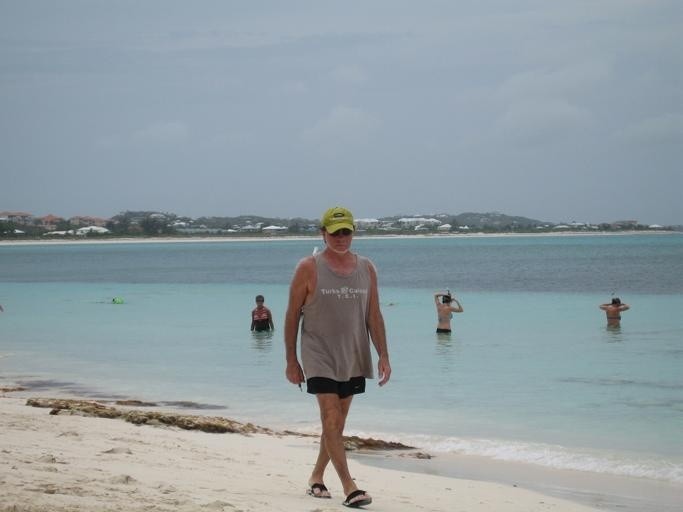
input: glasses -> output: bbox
[329,229,352,237]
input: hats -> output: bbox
[321,207,354,234]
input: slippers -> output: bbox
[306,483,331,498]
[342,490,371,507]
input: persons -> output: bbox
[433,286,464,339]
[285,206,392,507]
[250,295,275,337]
[599,297,630,333]
